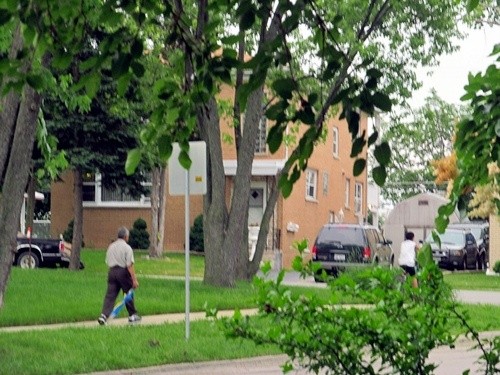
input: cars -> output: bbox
[426,230,478,271]
[12,235,65,268]
[445,221,489,271]
[311,225,394,283]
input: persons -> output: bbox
[97,226,141,325]
[399,232,423,287]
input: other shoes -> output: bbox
[128,314,142,325]
[97,313,106,325]
